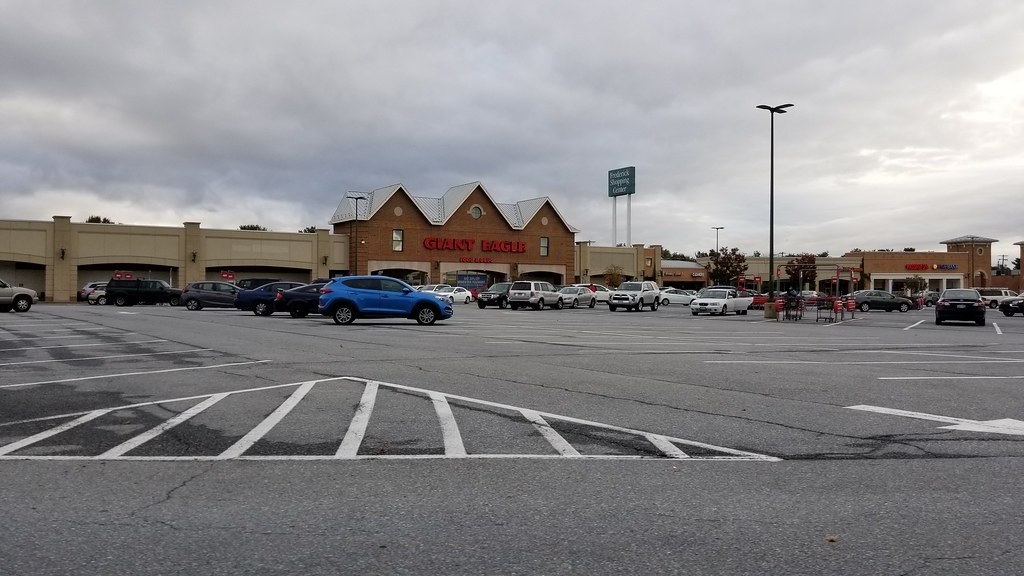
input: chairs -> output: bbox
[201,284,204,289]
[211,284,218,291]
[151,282,156,288]
[869,293,873,296]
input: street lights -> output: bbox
[347,196,366,276]
[757,104,794,318]
[967,236,981,288]
[712,227,724,285]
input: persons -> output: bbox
[786,287,799,319]
[590,284,595,292]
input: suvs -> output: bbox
[180,281,244,310]
[0,279,39,312]
[310,275,453,325]
[81,281,108,305]
[508,281,563,310]
[608,281,660,311]
[477,282,513,309]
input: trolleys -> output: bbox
[816,296,834,323]
[782,294,803,321]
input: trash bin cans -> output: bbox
[765,303,776,318]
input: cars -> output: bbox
[999,292,1024,316]
[743,289,820,297]
[839,290,913,312]
[273,283,326,318]
[553,285,598,308]
[659,285,754,316]
[234,282,309,317]
[915,290,942,307]
[935,289,986,326]
[412,284,488,304]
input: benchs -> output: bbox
[752,296,841,310]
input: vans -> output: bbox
[570,284,612,305]
[238,278,281,289]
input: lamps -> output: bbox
[323,255,328,265]
[192,251,197,263]
[583,269,589,277]
[658,270,664,277]
[640,270,645,277]
[60,247,66,260]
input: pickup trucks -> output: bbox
[106,278,183,306]
[981,290,1018,309]
